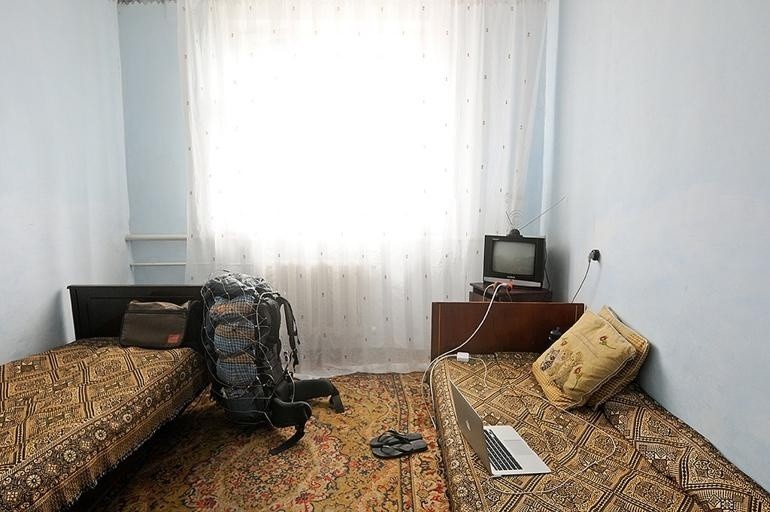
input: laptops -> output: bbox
[448,378,552,478]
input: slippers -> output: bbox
[370,429,428,459]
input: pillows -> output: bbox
[533,311,636,406]
[589,305,650,411]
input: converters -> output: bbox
[457,351,470,363]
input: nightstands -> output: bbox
[469,283,552,302]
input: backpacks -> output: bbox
[200,271,334,431]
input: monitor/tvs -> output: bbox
[483,235,546,288]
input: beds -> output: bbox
[429,300,769,512]
[1,285,214,511]
